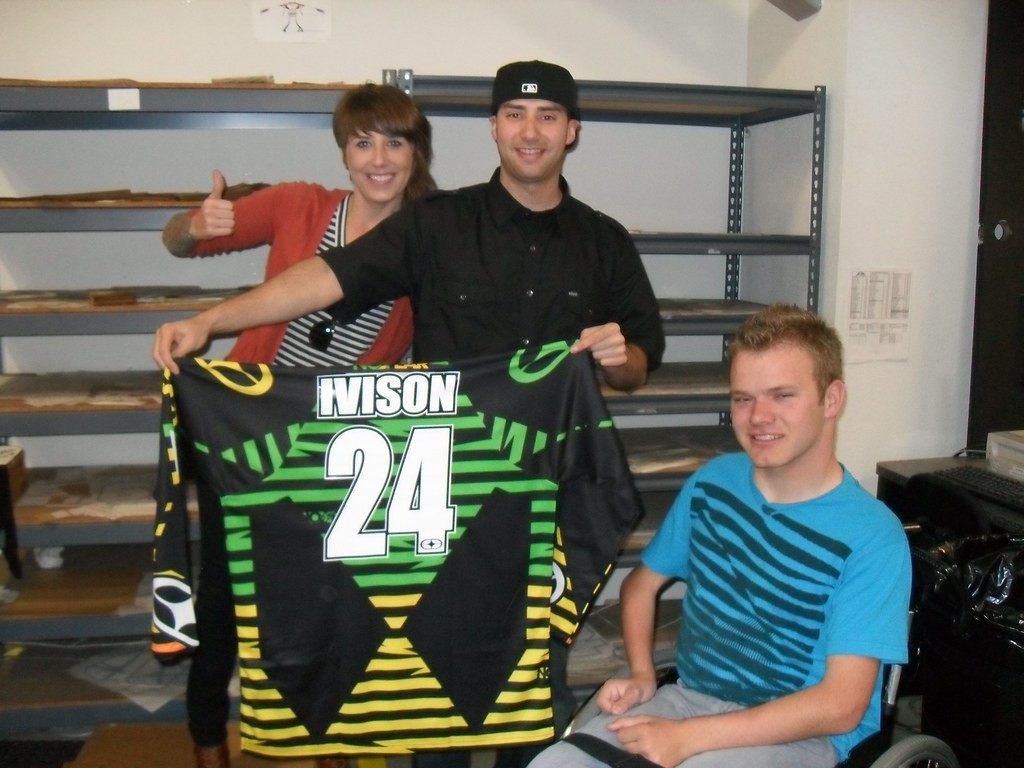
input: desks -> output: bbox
[874,456,1024,768]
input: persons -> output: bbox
[527,303,912,768]
[161,83,439,768]
[155,59,667,768]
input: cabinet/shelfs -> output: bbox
[0,73,827,754]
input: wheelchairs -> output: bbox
[603,522,950,768]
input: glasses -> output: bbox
[309,314,357,352]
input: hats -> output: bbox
[491,59,579,152]
